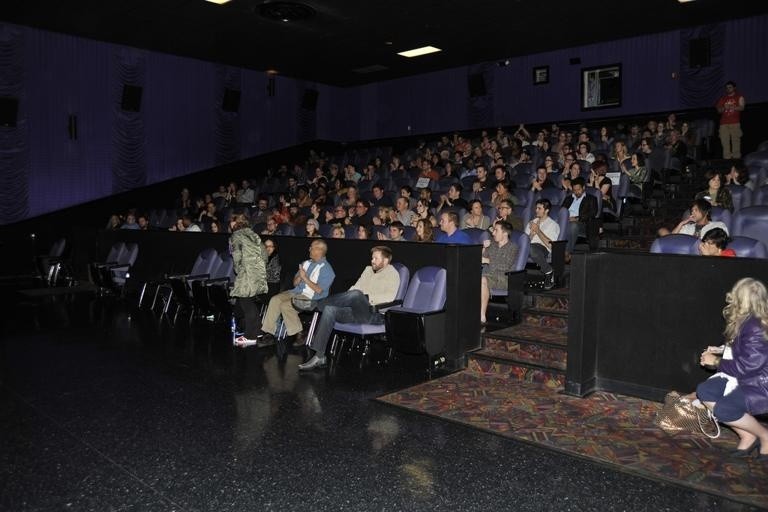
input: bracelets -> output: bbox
[713,357,722,367]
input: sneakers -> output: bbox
[544,271,554,290]
[257,334,275,347]
[292,332,307,347]
[235,336,257,342]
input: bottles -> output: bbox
[685,166,690,174]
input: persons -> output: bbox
[109,114,766,372]
[717,82,746,161]
[360,406,409,453]
[694,277,768,460]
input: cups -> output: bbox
[703,196,712,203]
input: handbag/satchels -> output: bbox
[654,390,721,439]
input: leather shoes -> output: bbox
[291,297,315,312]
[298,356,328,370]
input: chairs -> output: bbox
[29,124,766,422]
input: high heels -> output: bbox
[729,437,767,463]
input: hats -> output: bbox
[287,203,298,208]
[296,185,309,193]
